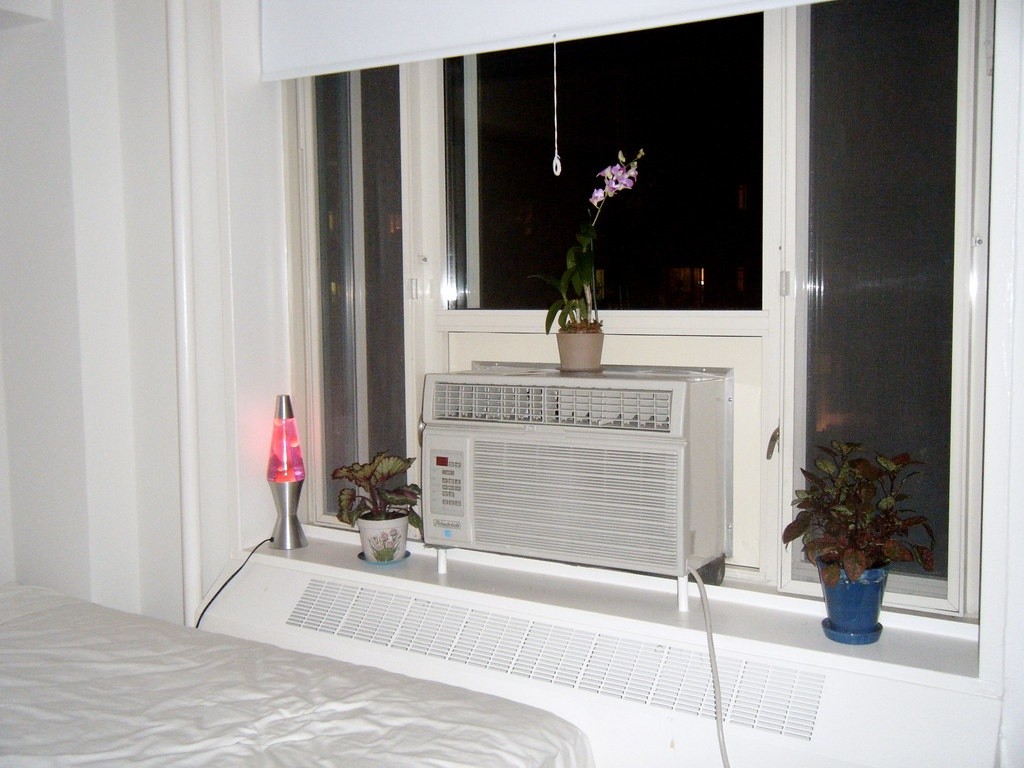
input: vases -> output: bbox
[556,332,604,376]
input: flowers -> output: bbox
[525,148,645,336]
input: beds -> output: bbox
[0,579,594,768]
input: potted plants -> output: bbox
[332,449,424,570]
[780,440,934,646]
